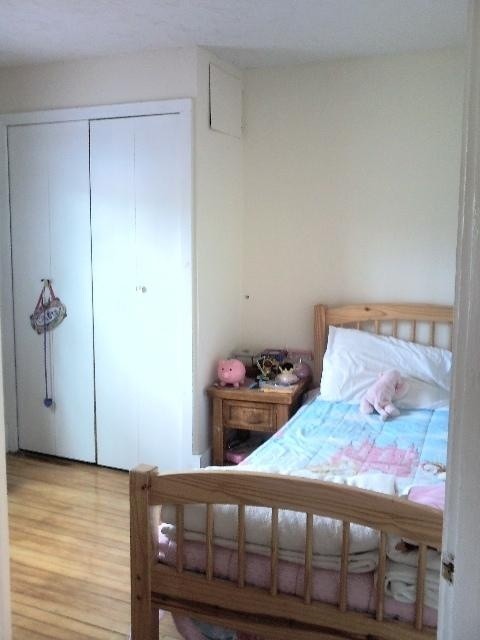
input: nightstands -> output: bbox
[206,376,313,466]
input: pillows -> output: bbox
[318,324,453,411]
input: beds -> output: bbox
[128,301,455,640]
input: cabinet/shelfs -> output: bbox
[0,97,193,478]
